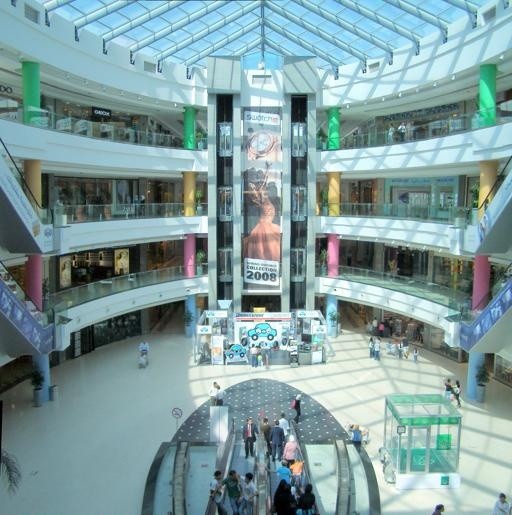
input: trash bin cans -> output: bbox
[48,384,59,401]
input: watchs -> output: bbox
[246,130,282,163]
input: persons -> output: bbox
[347,423,369,445]
[368,316,424,361]
[445,379,461,408]
[141,341,149,366]
[492,493,511,515]
[244,160,280,261]
[385,116,416,144]
[209,381,316,515]
[432,504,444,515]
[251,341,271,367]
